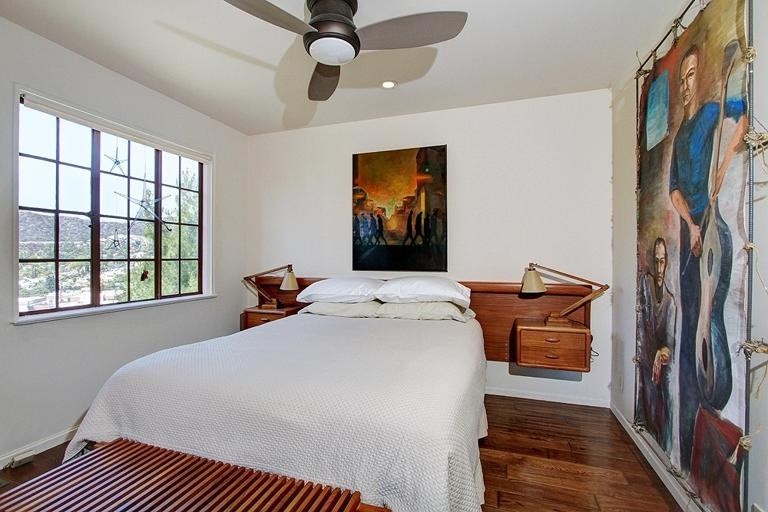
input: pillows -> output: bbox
[376,302,476,323]
[374,276,471,309]
[295,277,386,302]
[297,302,381,318]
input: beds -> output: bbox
[62,276,591,512]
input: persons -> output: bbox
[402,208,447,245]
[634,237,678,456]
[353,212,388,245]
[669,47,748,478]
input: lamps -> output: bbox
[521,264,609,326]
[240,265,299,309]
[308,36,356,66]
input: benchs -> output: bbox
[1,437,391,512]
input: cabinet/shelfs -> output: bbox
[240,305,302,331]
[515,318,591,372]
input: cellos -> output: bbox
[694,39,740,412]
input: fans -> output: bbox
[224,0,467,101]
